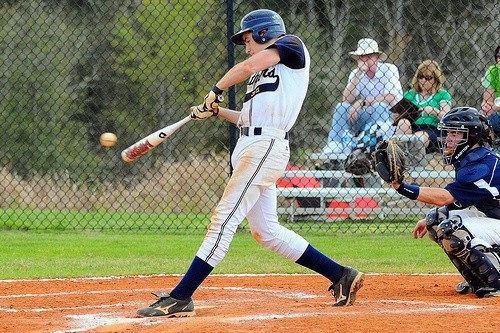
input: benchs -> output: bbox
[276,153,456,221]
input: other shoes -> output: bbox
[314,160,342,172]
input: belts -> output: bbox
[235,127,290,140]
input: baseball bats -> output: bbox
[121,95,225,163]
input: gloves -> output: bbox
[202,85,224,113]
[190,103,220,120]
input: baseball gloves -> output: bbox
[373,139,406,185]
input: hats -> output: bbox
[348,38,388,62]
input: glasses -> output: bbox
[417,73,435,81]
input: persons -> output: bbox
[481,45,500,138]
[390,59,452,155]
[136,8,366,319]
[316,38,404,171]
[374,106,500,298]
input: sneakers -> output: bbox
[328,265,366,307]
[136,291,196,318]
[455,283,500,298]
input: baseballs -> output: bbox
[100,133,118,148]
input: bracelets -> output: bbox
[396,182,420,201]
[363,101,366,106]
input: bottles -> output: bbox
[343,129,352,154]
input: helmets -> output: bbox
[230,8,287,44]
[437,106,488,165]
